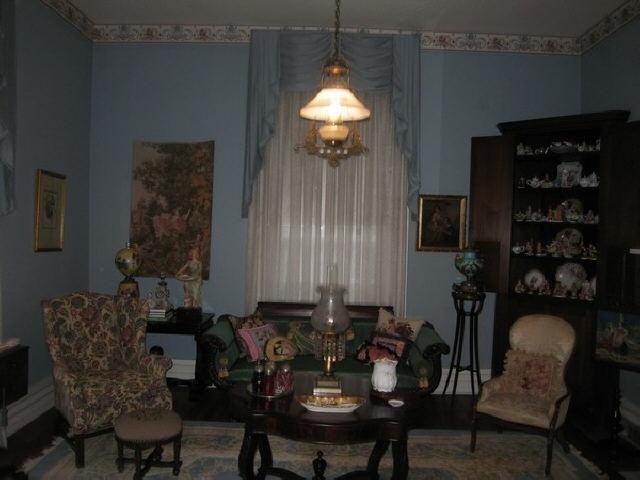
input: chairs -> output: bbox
[42,291,174,467]
[470,314,577,477]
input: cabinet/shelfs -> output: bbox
[469,110,640,404]
[441,292,485,407]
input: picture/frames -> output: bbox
[415,194,470,252]
[34,170,66,252]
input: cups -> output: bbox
[371,357,398,393]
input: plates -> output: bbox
[524,198,588,291]
[295,394,367,413]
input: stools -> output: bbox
[115,407,182,480]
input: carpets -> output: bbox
[17,420,610,480]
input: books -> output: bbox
[147,306,174,322]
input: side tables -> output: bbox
[146,312,215,402]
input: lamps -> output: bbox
[294,0,370,166]
[311,286,350,397]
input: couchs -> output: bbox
[201,301,450,410]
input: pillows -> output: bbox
[228,308,424,368]
[505,351,556,398]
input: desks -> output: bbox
[594,358,640,440]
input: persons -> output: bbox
[176,248,203,308]
[431,216,455,243]
[611,321,629,360]
[423,205,443,246]
